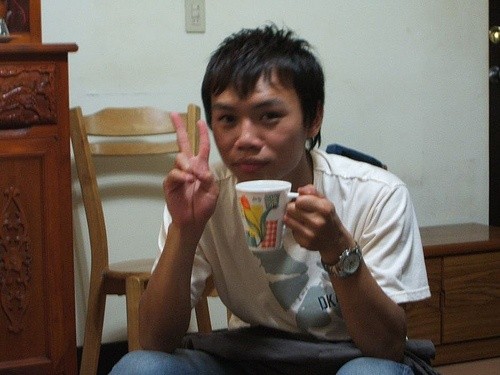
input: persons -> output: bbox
[105,21,441,375]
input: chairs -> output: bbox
[67,103,235,374]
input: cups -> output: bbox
[235,180,302,253]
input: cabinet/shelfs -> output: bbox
[404,223,498,366]
[1,41,79,374]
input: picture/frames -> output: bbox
[2,0,42,47]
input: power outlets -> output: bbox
[184,1,207,33]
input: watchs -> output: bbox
[319,241,363,280]
